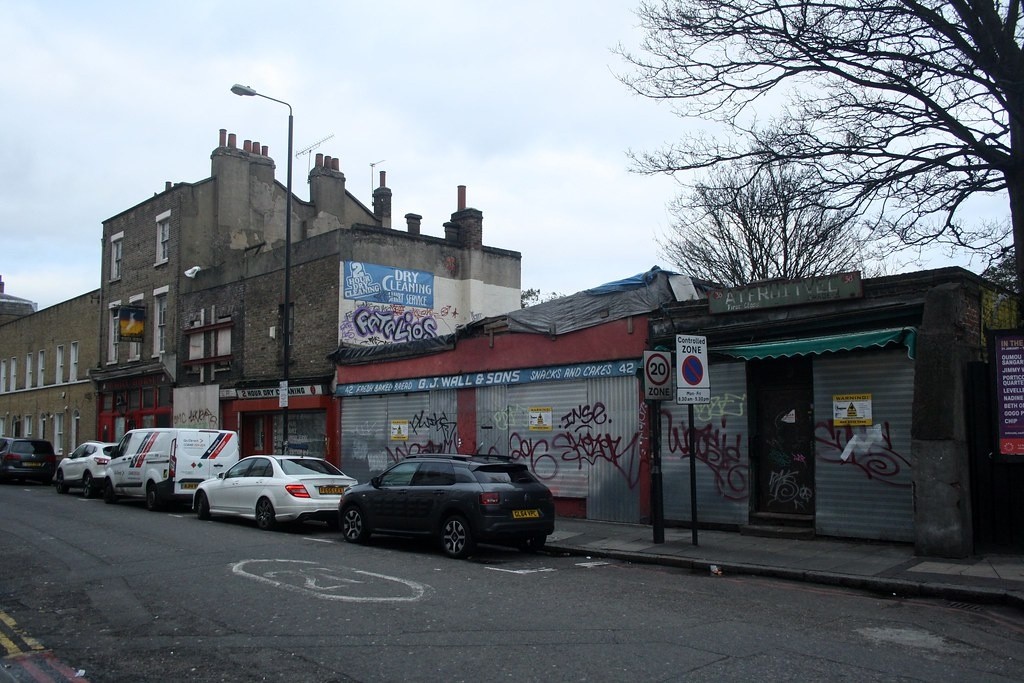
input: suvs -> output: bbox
[56,441,120,499]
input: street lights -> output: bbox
[230,84,294,455]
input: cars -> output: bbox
[338,453,555,559]
[192,455,359,530]
[0,437,56,486]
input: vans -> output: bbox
[102,428,239,511]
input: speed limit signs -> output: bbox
[646,353,670,385]
